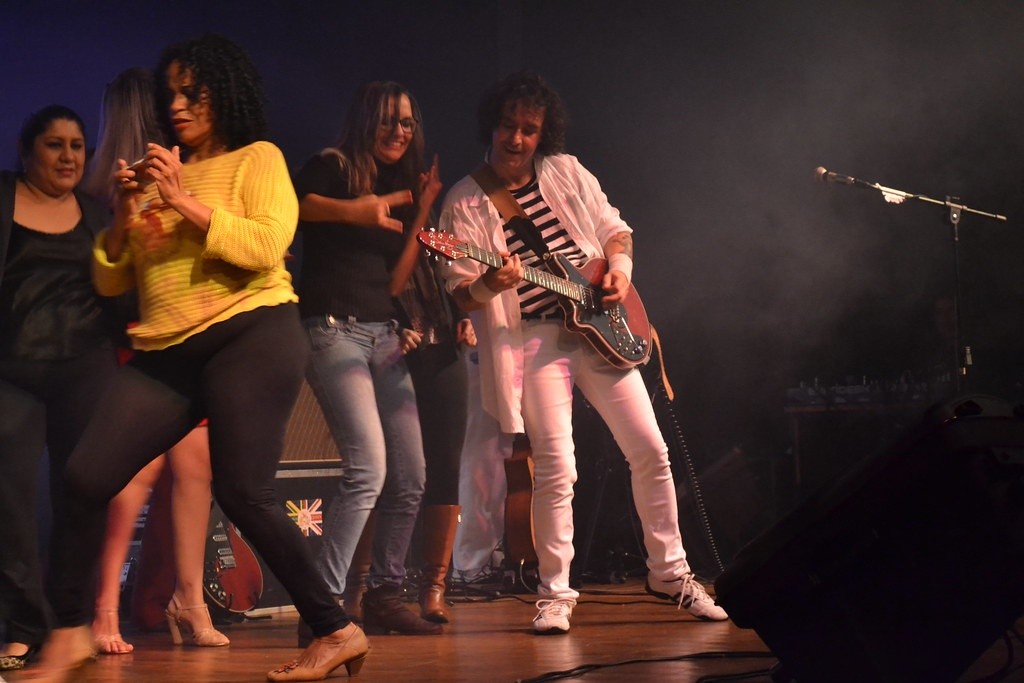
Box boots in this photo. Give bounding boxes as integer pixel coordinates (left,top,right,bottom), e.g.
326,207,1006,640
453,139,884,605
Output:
415,503,464,624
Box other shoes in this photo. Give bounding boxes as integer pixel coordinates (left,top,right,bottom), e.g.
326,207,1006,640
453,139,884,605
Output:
0,643,38,671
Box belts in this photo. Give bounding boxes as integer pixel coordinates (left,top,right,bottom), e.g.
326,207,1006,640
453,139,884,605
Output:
519,313,562,320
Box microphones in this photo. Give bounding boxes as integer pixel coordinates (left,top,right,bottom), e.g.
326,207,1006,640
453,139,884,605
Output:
814,167,855,184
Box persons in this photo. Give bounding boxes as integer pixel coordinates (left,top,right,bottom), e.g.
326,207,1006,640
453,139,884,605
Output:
294,81,448,632
437,70,730,632
1,105,120,668
344,266,479,621
449,346,514,592
0,35,368,682
82,67,228,652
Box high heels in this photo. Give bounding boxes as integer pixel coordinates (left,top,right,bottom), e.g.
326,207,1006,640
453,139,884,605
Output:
164,594,231,647
265,626,371,683
359,583,444,636
90,608,132,654
295,615,313,648
7,652,101,683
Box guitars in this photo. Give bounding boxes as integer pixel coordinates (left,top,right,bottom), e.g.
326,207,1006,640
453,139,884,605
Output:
416,225,651,372
202,517,265,614
504,433,537,565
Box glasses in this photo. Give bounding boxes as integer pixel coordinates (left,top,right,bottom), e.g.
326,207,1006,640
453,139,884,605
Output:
379,115,416,134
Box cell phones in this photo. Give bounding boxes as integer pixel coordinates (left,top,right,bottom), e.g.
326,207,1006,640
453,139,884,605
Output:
124,159,157,187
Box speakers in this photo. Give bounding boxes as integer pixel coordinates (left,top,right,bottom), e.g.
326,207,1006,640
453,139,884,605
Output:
713,392,1024,683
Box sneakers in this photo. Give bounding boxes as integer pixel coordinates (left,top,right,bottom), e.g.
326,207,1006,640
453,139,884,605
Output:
532,596,578,634
644,569,729,621
